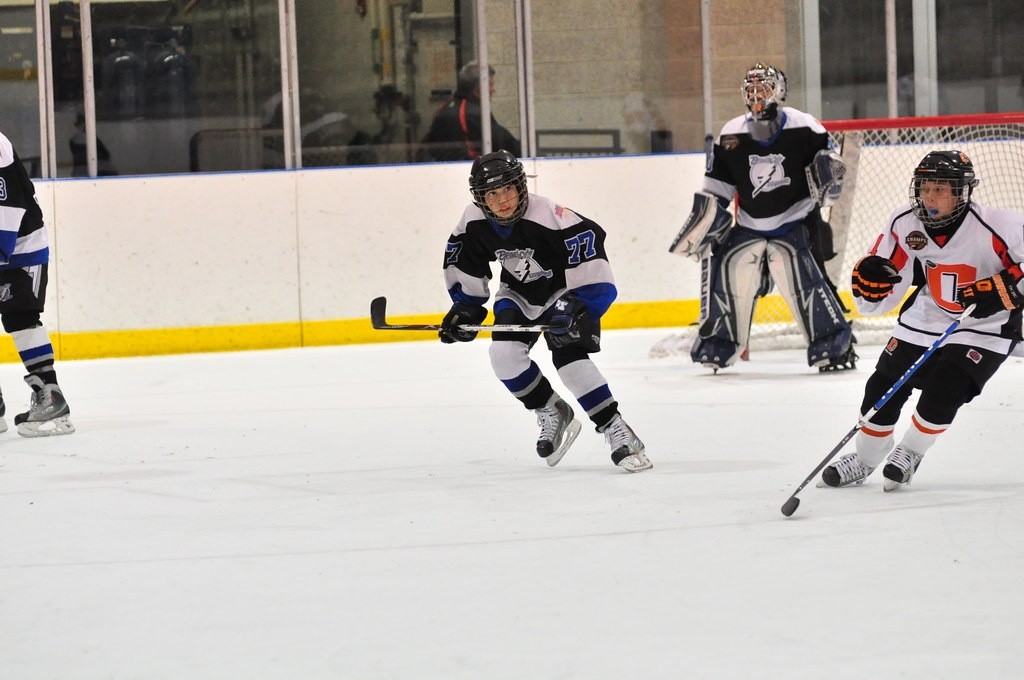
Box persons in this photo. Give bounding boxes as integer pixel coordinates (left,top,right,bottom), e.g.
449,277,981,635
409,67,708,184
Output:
66,112,121,178
349,82,420,165
438,148,652,471
0,129,75,438
815,150,1024,493
415,60,528,161
668,62,859,374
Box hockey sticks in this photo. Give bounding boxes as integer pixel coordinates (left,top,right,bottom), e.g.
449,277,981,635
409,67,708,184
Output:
366,292,553,335
781,304,976,513
686,133,716,328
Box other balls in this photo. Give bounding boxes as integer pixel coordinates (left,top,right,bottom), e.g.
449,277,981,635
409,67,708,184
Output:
783,498,801,517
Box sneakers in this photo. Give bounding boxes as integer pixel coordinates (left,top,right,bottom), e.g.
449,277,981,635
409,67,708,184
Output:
816,453,877,489
14,370,76,437
0,388,8,433
535,391,582,466
595,411,653,472
882,441,924,493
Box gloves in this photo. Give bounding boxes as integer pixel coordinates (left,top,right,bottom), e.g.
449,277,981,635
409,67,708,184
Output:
852,256,902,303
545,295,588,351
438,301,488,343
957,270,1024,319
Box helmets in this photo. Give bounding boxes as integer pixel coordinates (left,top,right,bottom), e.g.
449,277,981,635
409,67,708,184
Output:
914,150,979,228
469,149,529,226
742,61,788,118
